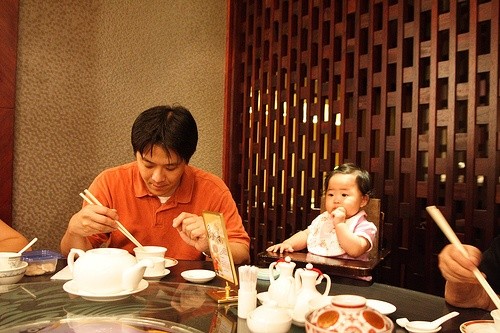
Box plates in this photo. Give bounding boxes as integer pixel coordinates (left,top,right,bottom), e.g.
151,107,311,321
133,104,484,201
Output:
405,321,442,333
256,291,267,305
63,279,149,302
459,320,500,333
142,256,178,280
181,269,216,284
327,296,396,315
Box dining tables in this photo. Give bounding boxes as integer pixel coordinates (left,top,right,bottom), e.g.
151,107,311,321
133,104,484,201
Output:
0,260,494,333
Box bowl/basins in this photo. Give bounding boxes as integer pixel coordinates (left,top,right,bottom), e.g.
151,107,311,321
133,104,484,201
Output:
246,311,293,333
257,268,278,287
0,251,28,285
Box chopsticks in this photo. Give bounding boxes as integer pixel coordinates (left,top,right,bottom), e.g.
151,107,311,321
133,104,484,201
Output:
426,206,500,311
79,189,145,249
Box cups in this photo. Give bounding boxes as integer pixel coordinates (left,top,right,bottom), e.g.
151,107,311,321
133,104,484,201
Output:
237,318,251,333
490,309,500,333
304,294,394,333
132,246,167,275
237,289,257,319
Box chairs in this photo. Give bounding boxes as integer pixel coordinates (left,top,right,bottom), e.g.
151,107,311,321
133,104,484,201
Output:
257,195,390,282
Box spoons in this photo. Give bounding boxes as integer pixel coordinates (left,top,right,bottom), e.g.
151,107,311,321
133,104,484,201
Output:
406,311,459,331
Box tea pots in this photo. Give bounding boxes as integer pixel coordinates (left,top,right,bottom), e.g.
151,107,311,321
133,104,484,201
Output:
266,256,331,321
67,248,154,295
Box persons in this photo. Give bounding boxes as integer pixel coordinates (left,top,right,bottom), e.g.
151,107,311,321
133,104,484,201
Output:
438,243,500,312
266,163,378,281
60,105,250,262
0,218,32,255
